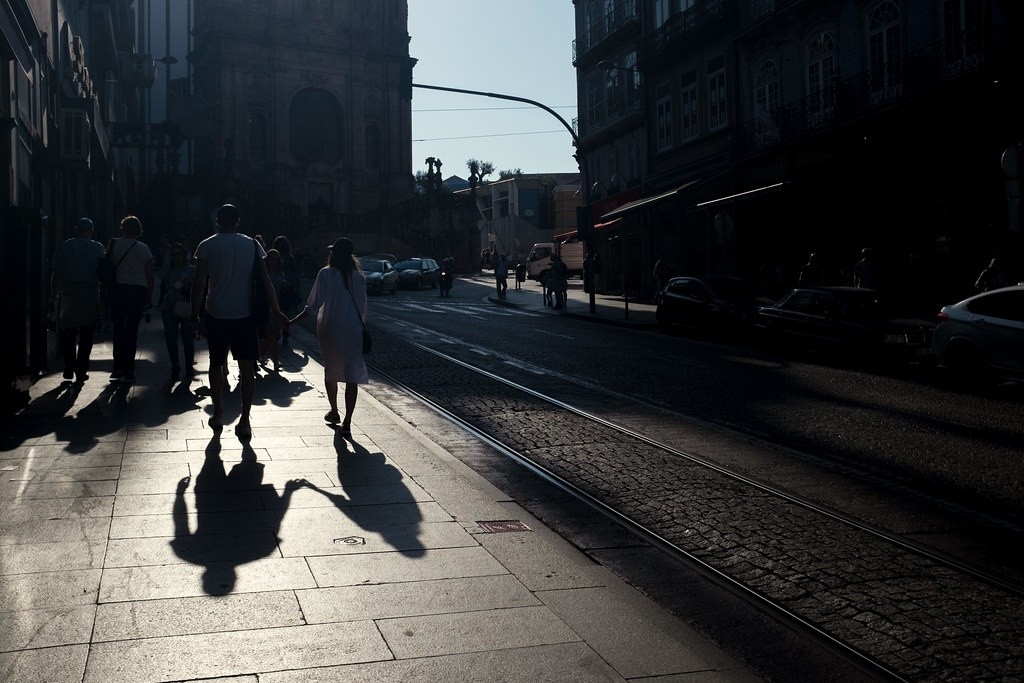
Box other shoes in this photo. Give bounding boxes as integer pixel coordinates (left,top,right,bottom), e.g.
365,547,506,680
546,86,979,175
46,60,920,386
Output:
324,411,341,423
553,304,562,310
185,368,200,377
171,367,181,380
63,370,73,379
208,417,223,431
76,374,89,381
274,364,283,370
235,424,251,444
340,426,351,436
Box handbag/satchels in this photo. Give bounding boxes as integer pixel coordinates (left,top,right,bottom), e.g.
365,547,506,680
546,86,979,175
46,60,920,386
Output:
252,239,270,314
362,328,372,355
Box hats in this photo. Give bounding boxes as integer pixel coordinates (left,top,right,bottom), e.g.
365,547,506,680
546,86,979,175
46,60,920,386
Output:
215,204,240,222
77,217,94,231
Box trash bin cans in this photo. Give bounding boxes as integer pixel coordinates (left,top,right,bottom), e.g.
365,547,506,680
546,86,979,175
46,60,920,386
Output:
516,264,526,282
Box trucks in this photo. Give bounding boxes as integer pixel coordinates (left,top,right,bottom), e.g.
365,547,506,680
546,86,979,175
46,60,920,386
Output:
523,236,584,284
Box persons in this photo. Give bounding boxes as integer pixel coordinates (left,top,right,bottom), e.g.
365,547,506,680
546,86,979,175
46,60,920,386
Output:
436,247,602,310
654,253,675,294
974,256,1004,293
51,203,369,436
799,250,821,292
853,248,876,289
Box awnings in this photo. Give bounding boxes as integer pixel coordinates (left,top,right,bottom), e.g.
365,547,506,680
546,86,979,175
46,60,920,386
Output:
550,171,794,242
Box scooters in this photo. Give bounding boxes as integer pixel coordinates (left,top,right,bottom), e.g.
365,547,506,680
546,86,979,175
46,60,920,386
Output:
434,267,454,297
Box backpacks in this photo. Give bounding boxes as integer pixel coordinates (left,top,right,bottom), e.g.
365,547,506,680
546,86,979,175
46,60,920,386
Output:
96,238,138,293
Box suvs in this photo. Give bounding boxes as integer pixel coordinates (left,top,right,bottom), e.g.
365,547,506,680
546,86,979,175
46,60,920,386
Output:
931,279,1024,392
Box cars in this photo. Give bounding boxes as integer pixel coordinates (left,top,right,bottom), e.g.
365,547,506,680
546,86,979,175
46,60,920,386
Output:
656,273,777,345
368,253,399,266
357,259,401,295
748,286,938,381
394,255,440,291
